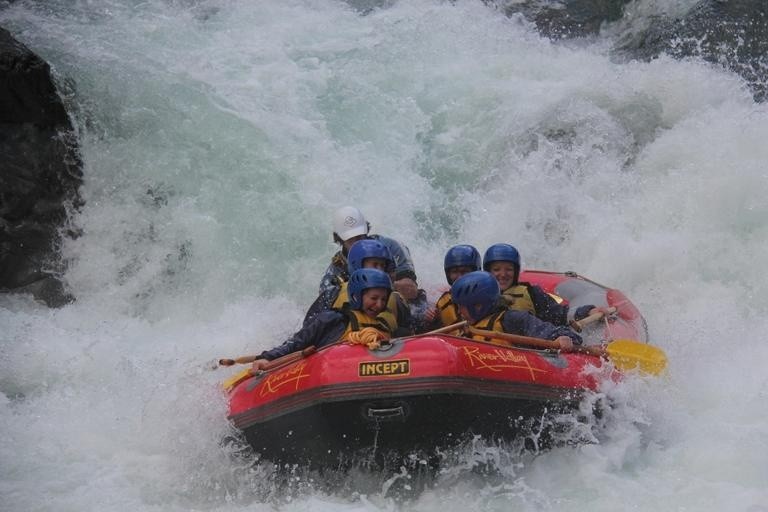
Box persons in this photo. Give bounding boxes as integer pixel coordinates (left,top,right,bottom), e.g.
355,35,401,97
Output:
318,207,418,301
483,243,608,326
414,244,481,338
251,267,417,375
441,271,582,352
302,239,426,328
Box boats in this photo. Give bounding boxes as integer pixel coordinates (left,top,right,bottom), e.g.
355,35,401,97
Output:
223,269,648,498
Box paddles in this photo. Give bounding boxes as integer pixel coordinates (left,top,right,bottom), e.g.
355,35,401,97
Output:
569,289,640,332
222,346,316,390
464,325,666,377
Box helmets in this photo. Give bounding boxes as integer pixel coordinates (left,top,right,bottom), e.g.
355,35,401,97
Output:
445,245,482,286
450,271,500,320
348,268,393,310
483,243,521,284
348,239,395,272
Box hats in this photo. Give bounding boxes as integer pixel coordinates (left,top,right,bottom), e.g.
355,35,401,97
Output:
332,205,368,242
